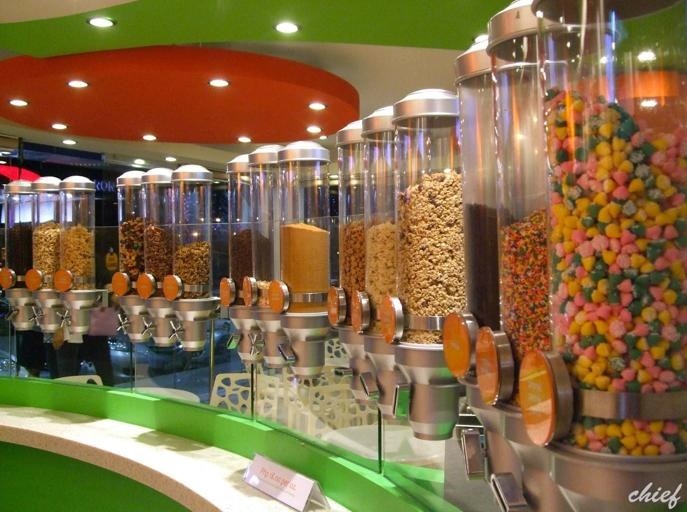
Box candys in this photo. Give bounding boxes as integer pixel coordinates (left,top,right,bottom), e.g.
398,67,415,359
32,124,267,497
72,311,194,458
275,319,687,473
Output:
498,85,687,457
